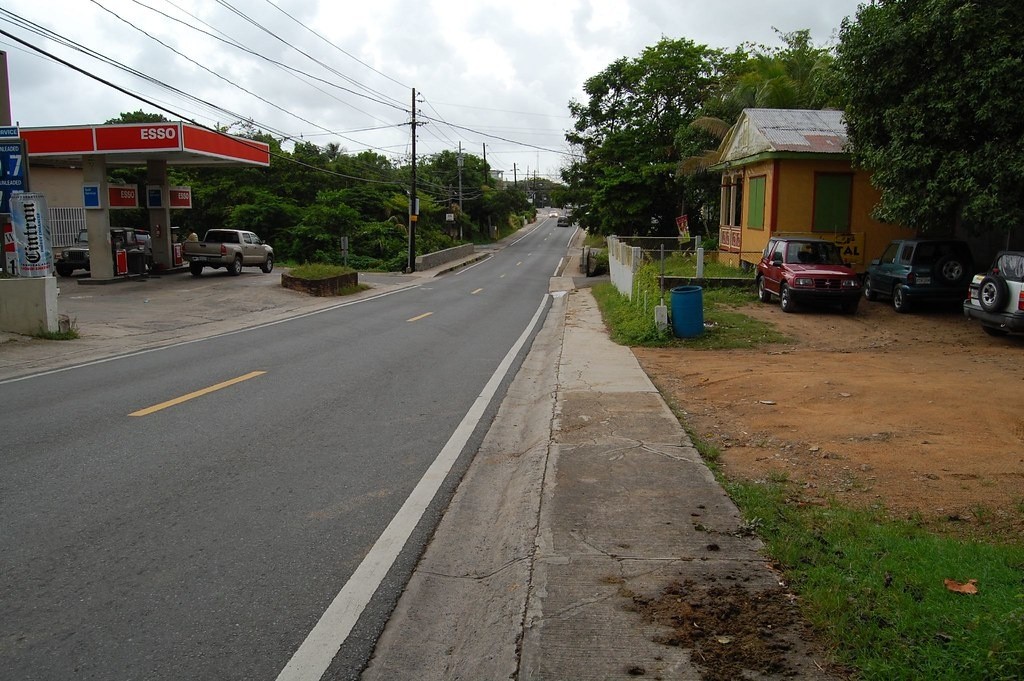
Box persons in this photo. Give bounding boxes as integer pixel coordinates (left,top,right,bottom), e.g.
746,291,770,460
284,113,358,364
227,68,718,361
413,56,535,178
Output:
144,237,153,269
186,228,198,242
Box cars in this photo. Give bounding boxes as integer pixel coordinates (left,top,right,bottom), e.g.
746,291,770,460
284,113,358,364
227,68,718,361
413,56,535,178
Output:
549,211,558,218
566,204,573,208
134,229,151,249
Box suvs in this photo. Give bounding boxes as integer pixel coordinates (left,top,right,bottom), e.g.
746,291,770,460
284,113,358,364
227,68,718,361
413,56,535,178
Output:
864,238,977,314
53,228,139,276
963,249,1024,336
755,237,864,311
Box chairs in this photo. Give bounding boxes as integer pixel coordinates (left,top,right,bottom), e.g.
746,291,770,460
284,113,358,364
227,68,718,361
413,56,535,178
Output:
798,251,814,264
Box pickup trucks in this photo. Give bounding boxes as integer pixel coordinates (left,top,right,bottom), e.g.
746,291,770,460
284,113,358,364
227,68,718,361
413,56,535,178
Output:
183,229,274,276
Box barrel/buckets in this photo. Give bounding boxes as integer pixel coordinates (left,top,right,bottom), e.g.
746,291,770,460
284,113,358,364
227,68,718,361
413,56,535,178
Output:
671,286,704,339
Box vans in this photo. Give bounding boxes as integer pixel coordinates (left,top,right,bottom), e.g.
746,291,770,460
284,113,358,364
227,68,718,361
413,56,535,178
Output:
557,216,570,227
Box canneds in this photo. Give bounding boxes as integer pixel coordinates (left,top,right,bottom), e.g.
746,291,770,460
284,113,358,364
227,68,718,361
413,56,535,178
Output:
8,192,54,278
